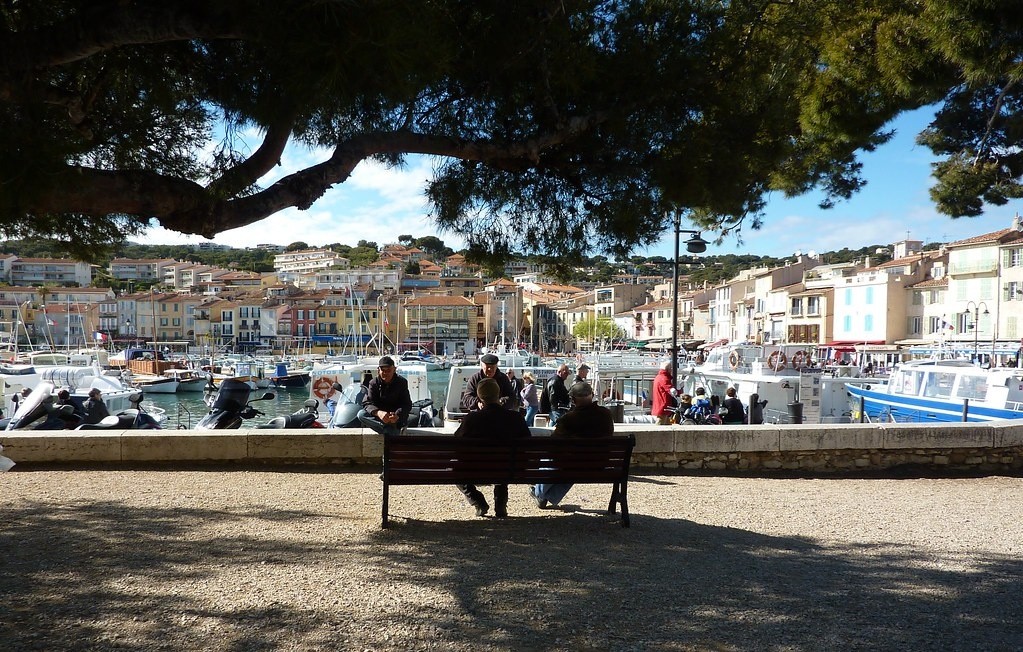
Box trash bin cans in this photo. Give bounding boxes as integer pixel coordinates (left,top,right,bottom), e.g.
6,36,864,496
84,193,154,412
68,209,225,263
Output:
787,402,802,423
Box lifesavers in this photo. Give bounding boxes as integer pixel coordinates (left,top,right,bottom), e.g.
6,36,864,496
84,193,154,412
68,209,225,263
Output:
314,377,336,398
602,389,620,400
728,351,739,370
767,350,787,371
576,354,582,361
792,350,810,371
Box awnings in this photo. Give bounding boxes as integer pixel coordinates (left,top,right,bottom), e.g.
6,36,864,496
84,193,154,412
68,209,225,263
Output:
612,339,728,351
817,341,1021,355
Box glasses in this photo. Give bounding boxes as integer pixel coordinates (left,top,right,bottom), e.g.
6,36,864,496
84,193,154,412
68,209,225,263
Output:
506,372,511,374
523,377,526,379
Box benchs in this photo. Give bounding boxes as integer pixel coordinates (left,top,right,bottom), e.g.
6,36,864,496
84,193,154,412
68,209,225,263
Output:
382,431,634,528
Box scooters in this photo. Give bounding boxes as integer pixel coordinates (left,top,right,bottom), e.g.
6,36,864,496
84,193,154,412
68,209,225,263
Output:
193,373,327,429
327,383,438,427
5,381,169,430
0,382,88,432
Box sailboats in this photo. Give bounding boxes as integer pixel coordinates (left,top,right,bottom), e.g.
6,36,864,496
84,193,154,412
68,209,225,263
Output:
0,271,1023,432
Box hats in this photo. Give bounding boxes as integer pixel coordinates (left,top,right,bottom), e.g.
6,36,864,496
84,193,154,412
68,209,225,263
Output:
727,388,737,397
481,354,499,364
58,390,69,399
557,364,571,373
575,363,591,369
567,381,592,397
379,356,394,366
696,387,706,395
478,378,500,398
88,387,101,397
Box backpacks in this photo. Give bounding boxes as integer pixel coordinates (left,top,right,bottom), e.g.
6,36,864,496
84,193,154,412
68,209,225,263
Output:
539,376,554,413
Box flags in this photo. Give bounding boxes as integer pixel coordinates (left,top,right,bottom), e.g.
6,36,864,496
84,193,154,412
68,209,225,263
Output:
46,315,59,326
942,320,954,330
92,330,108,341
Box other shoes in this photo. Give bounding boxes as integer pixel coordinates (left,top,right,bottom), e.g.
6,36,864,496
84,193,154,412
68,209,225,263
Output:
475,503,489,516
496,509,507,517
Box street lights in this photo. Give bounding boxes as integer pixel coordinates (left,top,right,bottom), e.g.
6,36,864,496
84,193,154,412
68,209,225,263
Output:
752,311,773,344
673,231,712,387
126,319,131,369
251,325,261,356
964,301,990,358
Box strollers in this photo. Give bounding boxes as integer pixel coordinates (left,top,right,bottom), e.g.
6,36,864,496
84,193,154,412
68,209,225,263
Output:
664,395,722,425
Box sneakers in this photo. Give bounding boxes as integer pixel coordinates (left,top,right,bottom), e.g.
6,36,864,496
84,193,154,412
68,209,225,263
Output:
529,486,547,509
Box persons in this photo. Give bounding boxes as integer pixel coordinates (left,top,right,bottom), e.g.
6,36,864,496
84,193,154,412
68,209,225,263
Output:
973,355,1018,368
811,358,871,373
453,349,478,359
539,364,571,428
0,388,110,424
360,370,373,389
463,354,516,412
699,352,704,362
357,356,412,481
520,372,538,428
674,387,768,424
570,363,591,412
447,378,531,518
529,382,614,508
506,368,522,412
651,362,684,425
323,398,338,428
